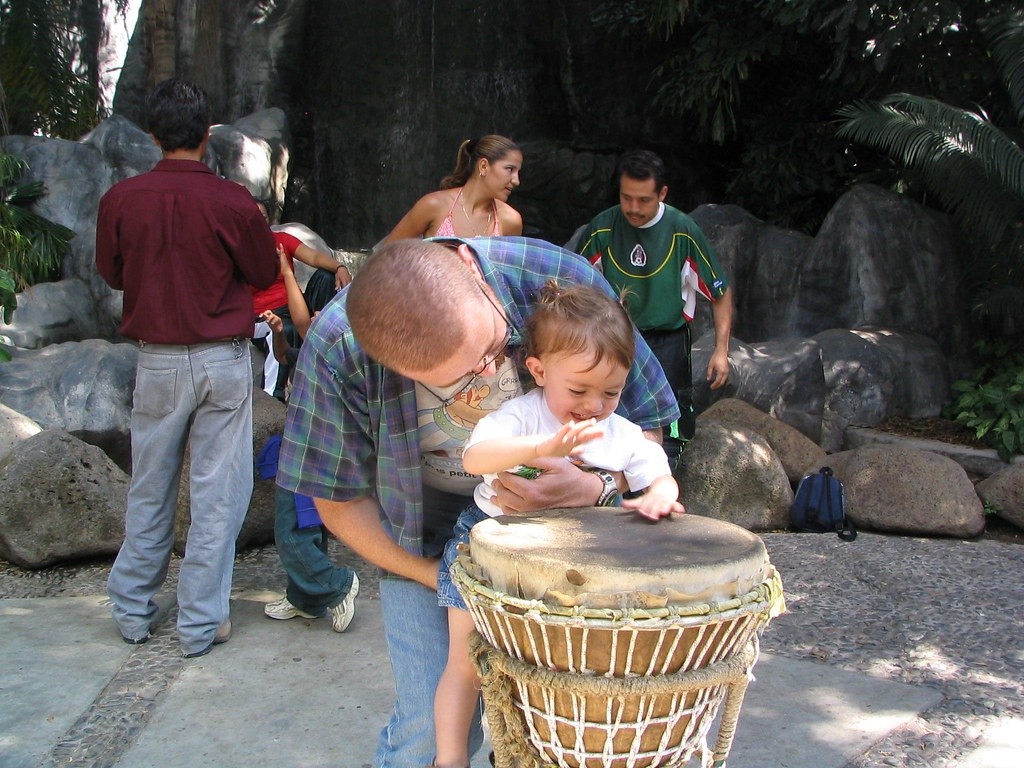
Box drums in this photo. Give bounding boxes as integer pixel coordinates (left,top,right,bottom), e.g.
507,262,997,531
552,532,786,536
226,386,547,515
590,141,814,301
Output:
447,506,781,767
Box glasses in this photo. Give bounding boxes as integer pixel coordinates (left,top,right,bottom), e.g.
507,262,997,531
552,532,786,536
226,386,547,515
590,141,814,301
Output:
416,276,514,406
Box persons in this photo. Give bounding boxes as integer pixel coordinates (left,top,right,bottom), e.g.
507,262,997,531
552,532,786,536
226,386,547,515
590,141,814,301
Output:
381,135,523,248
432,278,684,768
248,200,360,634
97,79,279,657
575,150,733,477
274,235,681,768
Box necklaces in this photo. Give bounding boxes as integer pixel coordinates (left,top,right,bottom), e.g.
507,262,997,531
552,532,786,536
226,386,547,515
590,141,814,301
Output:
461,190,491,238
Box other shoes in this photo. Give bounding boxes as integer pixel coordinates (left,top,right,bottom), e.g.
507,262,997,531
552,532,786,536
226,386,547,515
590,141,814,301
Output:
184,619,232,659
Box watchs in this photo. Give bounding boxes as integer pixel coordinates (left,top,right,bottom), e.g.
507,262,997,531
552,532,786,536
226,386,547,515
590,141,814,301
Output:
588,468,618,507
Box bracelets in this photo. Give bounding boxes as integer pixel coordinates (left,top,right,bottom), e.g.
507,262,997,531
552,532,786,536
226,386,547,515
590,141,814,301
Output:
337,265,346,271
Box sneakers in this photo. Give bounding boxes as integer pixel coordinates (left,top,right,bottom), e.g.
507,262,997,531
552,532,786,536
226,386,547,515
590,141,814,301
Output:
327,571,359,634
264,598,317,619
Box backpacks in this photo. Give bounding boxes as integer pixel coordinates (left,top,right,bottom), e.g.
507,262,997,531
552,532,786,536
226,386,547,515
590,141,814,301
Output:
790,466,857,542
